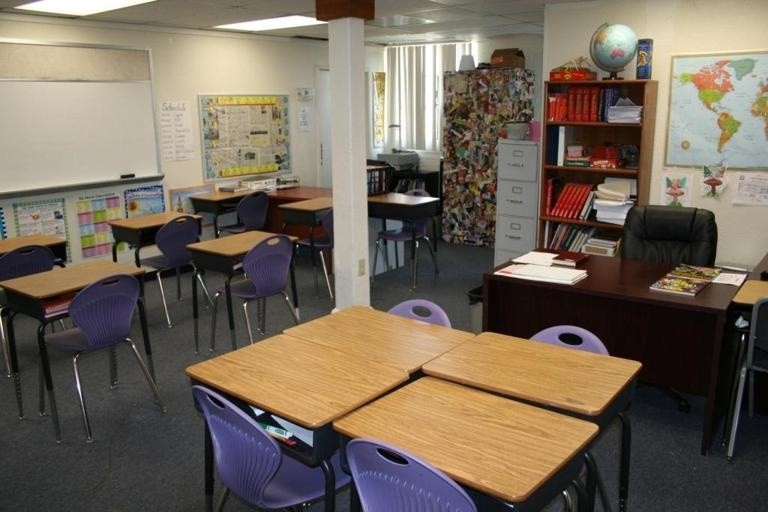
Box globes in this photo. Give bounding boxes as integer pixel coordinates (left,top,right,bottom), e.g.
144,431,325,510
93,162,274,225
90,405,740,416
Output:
589,21,638,80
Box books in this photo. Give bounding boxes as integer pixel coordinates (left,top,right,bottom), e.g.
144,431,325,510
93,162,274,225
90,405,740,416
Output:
552,250,590,267
42,308,69,320
218,174,302,193
648,274,711,296
666,260,723,283
40,291,79,315
367,167,427,197
492,262,588,285
541,83,644,259
513,264,587,282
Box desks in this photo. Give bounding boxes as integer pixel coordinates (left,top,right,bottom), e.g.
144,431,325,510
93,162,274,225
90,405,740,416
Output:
481,250,748,455
732,280,768,308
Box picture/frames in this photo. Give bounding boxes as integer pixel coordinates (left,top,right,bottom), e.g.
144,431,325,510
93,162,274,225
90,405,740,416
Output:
662,50,768,172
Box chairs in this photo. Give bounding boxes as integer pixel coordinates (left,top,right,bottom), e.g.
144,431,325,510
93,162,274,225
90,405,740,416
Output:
724,297,768,460
622,203,720,271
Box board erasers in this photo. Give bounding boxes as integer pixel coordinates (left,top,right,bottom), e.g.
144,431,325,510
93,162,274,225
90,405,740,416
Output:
121,173,134,179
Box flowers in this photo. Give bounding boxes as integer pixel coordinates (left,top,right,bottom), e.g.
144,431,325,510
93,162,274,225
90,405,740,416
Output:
513,90,535,119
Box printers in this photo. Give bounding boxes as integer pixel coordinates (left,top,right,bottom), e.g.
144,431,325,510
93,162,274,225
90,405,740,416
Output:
377,148,419,171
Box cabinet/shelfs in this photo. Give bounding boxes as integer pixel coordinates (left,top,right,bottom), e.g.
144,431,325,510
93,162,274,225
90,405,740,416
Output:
495,139,535,272
538,80,659,253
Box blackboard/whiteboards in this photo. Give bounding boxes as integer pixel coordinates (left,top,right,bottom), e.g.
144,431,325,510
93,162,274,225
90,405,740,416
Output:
0,79,164,199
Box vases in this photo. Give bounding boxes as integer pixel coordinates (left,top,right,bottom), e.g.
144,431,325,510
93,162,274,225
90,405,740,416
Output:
505,120,530,140
